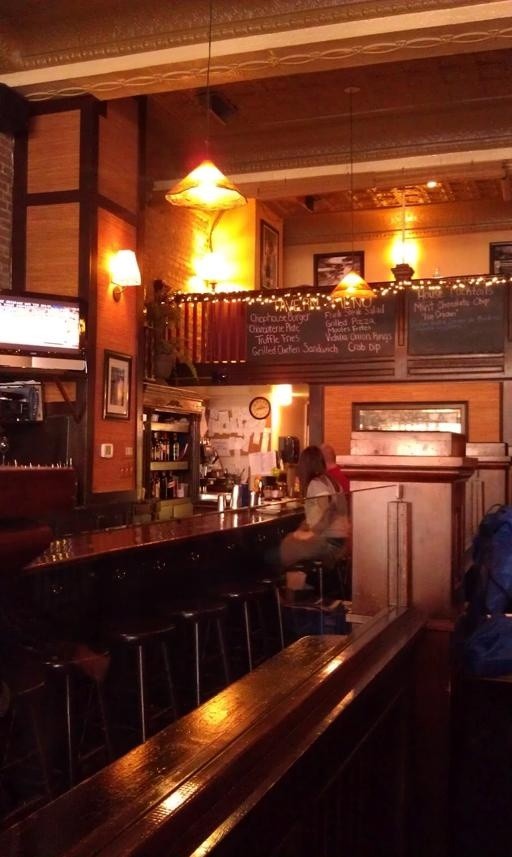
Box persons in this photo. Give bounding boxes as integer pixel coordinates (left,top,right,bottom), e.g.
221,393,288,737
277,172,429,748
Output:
276,445,348,566
319,443,350,493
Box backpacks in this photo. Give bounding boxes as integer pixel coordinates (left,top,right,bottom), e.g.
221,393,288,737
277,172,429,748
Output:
454,502,511,677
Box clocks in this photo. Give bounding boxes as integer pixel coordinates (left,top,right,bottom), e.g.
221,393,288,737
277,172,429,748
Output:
248,396,271,418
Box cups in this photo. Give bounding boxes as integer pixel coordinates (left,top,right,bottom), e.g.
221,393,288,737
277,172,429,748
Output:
215,481,257,513
264,484,281,498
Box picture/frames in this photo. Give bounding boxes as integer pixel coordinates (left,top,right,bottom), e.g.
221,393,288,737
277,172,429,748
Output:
488,240,511,276
312,250,366,286
102,348,133,422
259,219,281,290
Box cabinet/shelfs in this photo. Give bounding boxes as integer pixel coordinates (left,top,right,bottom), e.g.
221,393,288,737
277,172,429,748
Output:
139,381,204,523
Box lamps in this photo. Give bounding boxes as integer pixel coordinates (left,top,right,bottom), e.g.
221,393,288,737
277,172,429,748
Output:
329,86,379,301
163,2,249,214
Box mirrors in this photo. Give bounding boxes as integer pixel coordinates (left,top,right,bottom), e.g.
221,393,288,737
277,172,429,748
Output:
350,401,467,434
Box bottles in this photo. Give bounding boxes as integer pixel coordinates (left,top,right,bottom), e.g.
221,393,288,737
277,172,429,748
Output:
257,480,264,506
151,431,181,498
